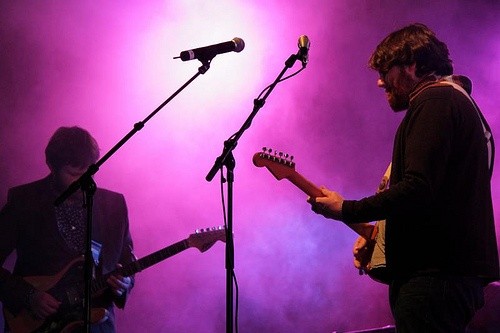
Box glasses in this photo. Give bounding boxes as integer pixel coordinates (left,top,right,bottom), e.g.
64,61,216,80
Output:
379,61,396,81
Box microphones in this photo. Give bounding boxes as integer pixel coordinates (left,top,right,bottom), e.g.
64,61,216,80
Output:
298,35,311,67
172,37,245,63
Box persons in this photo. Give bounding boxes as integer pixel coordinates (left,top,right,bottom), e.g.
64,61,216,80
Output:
1,125,137,333
307,23,500,333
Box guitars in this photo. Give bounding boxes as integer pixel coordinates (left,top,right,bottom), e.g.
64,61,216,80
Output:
253,147,392,285
2,225,233,333
